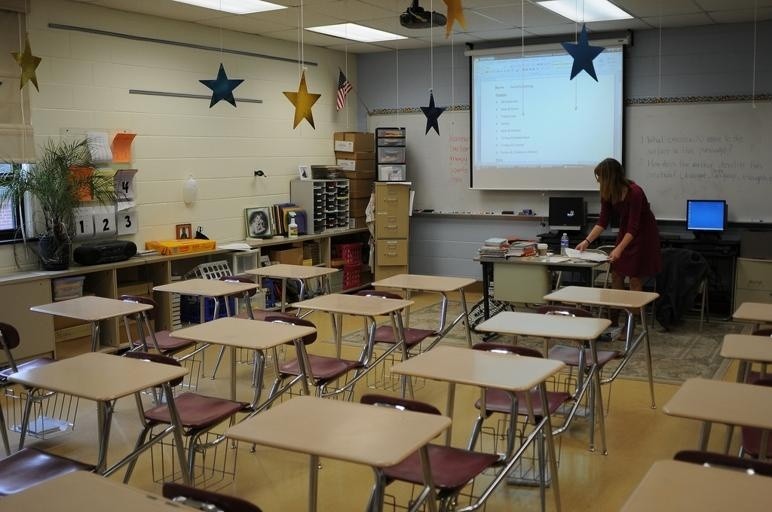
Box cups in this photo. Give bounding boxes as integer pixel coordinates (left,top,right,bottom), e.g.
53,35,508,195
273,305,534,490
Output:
538,244,548,257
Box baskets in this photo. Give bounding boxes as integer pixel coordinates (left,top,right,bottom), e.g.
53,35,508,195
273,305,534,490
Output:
341,265,362,290
183,260,236,306
334,242,363,266
180,297,237,323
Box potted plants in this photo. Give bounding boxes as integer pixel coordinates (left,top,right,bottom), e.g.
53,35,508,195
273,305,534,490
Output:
0,139,119,273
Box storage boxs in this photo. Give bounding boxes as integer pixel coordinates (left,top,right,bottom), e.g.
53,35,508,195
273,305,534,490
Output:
272,246,305,267
334,128,377,226
53,274,159,359
378,124,408,185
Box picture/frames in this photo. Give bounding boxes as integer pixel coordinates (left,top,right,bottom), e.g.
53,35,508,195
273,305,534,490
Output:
176,223,192,239
245,206,275,239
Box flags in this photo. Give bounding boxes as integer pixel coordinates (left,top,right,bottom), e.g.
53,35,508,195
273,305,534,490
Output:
335,71,352,112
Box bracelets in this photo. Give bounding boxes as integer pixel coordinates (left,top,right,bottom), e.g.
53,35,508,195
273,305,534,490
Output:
584,238,590,245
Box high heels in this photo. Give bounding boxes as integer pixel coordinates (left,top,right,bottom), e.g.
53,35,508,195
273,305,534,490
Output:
617,326,634,342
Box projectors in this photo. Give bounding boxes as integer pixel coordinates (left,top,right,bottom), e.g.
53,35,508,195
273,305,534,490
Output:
400,12,447,29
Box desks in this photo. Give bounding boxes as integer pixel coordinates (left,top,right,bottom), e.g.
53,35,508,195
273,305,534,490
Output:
0,469,202,512
225,393,453,509
151,278,259,381
369,273,477,360
475,255,606,342
475,312,615,458
290,292,418,402
662,376,771,467
621,455,770,510
389,345,566,511
241,265,340,343
730,300,772,334
540,230,741,322
719,333,772,384
28,294,155,355
170,316,319,454
7,351,193,492
542,284,661,410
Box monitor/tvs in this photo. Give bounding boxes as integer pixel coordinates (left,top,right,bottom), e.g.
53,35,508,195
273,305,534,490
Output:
686,200,725,235
611,203,650,232
549,197,584,230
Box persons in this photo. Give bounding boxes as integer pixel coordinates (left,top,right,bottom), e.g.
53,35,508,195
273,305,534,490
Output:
249,212,266,236
180,227,188,238
575,158,663,341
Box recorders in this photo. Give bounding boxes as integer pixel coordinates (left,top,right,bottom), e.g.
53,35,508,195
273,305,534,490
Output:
74,241,137,265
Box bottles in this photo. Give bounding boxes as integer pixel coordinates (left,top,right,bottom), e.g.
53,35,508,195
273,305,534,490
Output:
288,218,299,238
560,233,569,257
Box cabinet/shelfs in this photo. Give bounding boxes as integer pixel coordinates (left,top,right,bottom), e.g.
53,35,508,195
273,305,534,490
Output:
331,228,373,290
735,256,772,324
291,179,349,231
115,260,169,344
373,183,414,281
170,245,260,334
260,235,331,296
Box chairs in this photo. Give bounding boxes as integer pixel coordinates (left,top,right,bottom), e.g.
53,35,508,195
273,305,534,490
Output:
118,295,200,386
1,446,100,497
123,351,252,487
353,390,504,512
0,319,62,455
162,480,260,512
537,303,632,416
670,446,770,475
211,276,278,382
249,315,365,453
648,241,715,333
346,290,440,401
466,341,574,488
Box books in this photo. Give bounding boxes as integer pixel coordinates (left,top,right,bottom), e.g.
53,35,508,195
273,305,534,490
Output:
481,238,537,258
